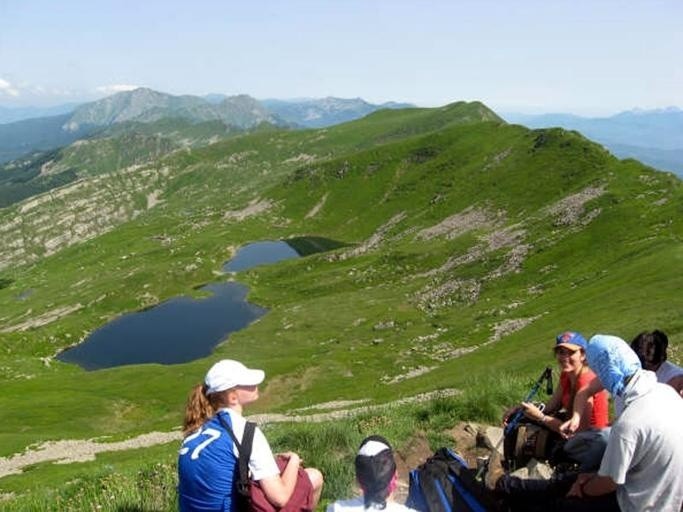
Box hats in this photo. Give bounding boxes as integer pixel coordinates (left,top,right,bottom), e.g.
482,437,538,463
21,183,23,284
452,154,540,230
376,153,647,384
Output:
354,434,397,491
553,331,589,354
204,359,266,396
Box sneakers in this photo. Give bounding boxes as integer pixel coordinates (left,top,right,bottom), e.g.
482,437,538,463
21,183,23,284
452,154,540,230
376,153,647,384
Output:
484,451,503,489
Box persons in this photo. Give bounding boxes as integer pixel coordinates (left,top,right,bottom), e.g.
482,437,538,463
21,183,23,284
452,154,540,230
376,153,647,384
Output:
325,435,417,512
479,334,683,511
557,326,682,444
176,357,324,512
501,330,611,472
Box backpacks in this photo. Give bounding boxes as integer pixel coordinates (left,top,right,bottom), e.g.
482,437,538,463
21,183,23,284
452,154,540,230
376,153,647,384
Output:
405,446,488,511
509,424,545,465
237,422,313,511
